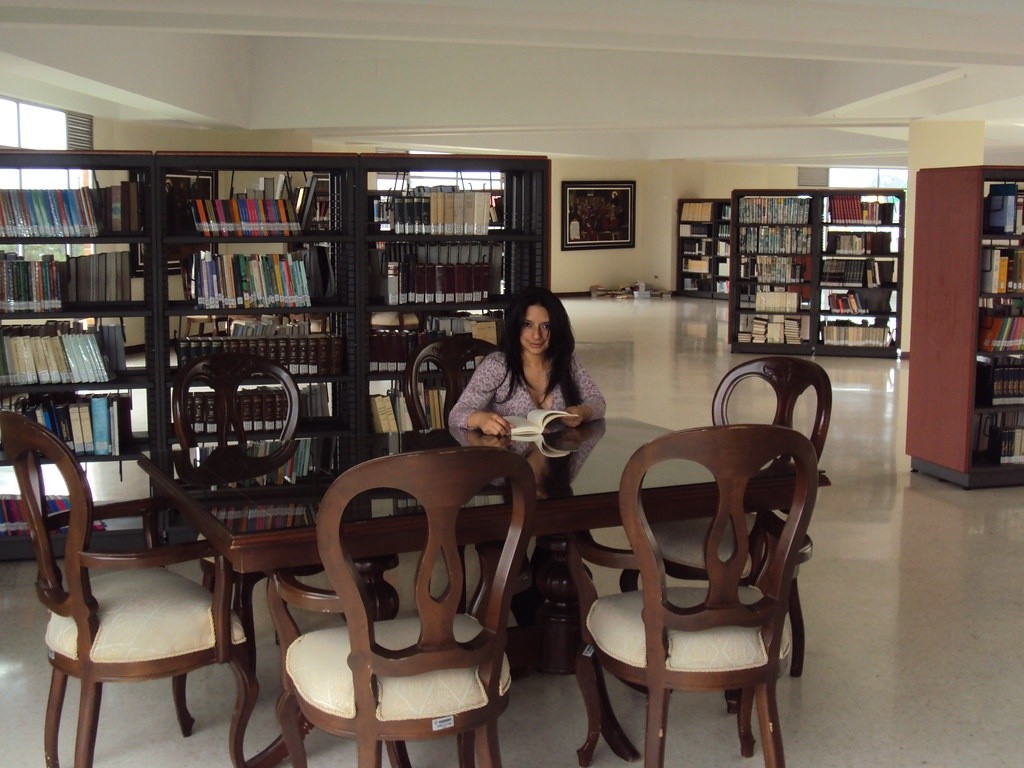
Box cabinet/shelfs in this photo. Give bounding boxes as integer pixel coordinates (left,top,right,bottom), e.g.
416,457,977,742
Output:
815,188,906,362
353,152,553,469
906,164,1024,490
676,197,714,297
0,150,157,561
730,188,817,355
155,149,355,539
714,197,731,300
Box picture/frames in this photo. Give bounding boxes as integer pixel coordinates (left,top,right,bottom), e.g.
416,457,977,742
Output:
560,180,635,251
128,168,219,278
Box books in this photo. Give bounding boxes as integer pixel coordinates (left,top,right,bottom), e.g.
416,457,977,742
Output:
0,321,128,386
737,198,814,344
0,493,106,537
821,193,899,350
0,250,133,314
371,184,506,520
0,180,148,237
972,184,1024,468
510,435,581,457
678,200,732,295
182,171,331,532
499,410,579,435
0,391,132,455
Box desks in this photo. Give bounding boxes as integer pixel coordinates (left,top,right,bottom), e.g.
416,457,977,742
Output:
136,419,833,768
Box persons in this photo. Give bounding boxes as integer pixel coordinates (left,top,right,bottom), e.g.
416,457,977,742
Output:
445,287,607,438
448,419,605,499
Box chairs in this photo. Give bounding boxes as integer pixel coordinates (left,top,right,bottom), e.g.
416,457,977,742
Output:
0,410,258,768
276,446,538,766
173,355,303,609
405,332,497,431
621,358,834,716
575,425,822,766
